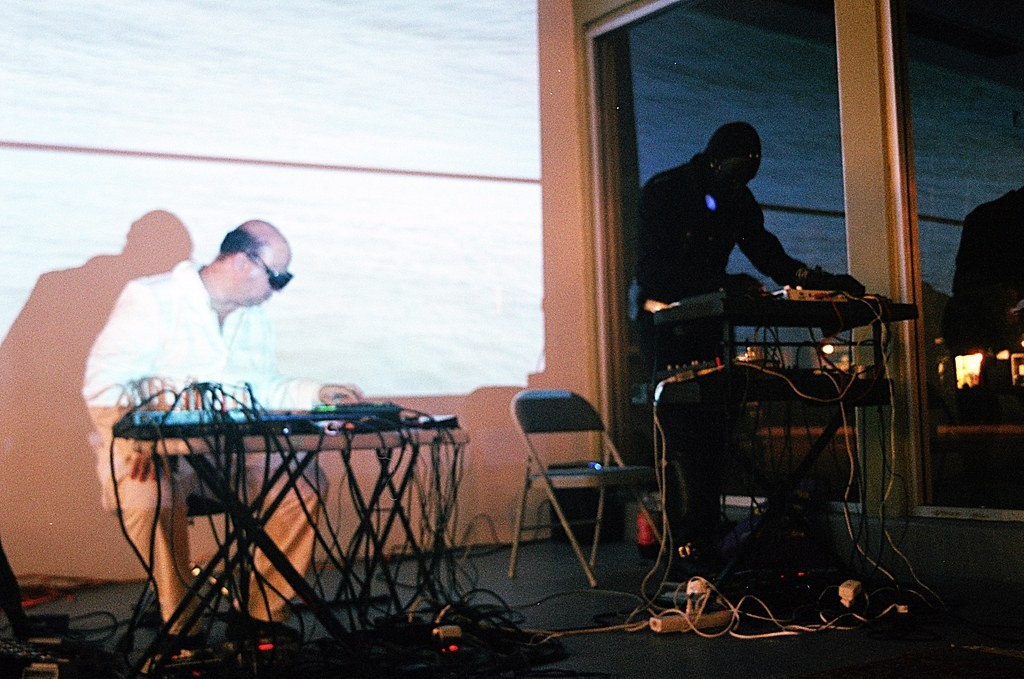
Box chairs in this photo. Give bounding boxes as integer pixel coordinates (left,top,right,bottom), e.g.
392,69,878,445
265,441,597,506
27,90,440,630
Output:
506,388,667,588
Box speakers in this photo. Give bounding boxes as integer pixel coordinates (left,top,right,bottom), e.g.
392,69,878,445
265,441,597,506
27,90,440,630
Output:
549,460,625,544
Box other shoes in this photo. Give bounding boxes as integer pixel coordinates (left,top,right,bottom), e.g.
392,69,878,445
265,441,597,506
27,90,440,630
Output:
226,615,299,646
179,630,214,659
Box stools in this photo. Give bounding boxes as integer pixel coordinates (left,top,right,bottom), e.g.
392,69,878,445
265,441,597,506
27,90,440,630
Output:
114,493,248,659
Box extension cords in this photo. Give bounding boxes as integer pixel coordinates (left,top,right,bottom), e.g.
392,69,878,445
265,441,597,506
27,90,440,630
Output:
649,610,731,633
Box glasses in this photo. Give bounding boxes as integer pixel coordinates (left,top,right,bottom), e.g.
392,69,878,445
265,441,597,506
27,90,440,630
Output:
247,252,293,291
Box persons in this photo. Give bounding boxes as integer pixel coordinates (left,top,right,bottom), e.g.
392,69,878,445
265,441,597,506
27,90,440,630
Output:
80,218,366,659
630,110,870,590
936,179,1024,507
